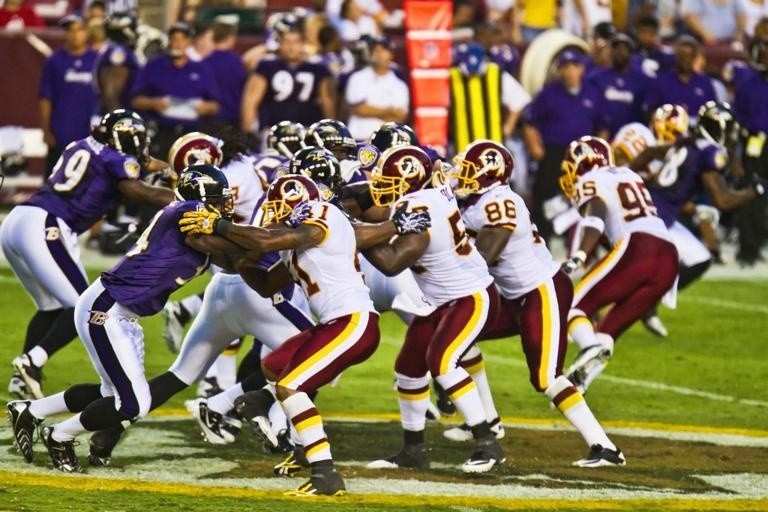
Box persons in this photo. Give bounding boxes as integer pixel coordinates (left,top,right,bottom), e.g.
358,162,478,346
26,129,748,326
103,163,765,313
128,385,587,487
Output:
1,1,768,494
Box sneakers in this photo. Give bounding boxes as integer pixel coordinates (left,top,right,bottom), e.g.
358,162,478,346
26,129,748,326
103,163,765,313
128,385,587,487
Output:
87,430,114,468
232,390,280,450
200,416,244,444
39,423,87,474
363,446,434,473
6,349,50,401
273,446,312,477
422,395,459,420
197,378,225,398
442,415,508,443
564,343,614,399
459,440,508,474
6,397,44,464
640,309,671,339
281,471,350,498
182,396,227,446
571,442,629,470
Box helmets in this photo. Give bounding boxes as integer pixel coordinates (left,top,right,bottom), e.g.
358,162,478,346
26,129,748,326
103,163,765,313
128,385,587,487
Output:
54,6,396,54
302,118,359,153
290,144,345,188
697,99,737,145
168,130,226,177
174,164,233,207
375,143,436,203
94,105,151,156
369,119,422,153
454,137,516,194
266,119,306,151
652,103,691,144
564,134,617,178
266,173,323,219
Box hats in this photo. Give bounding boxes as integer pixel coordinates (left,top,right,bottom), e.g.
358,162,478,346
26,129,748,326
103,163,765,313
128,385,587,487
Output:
556,23,698,67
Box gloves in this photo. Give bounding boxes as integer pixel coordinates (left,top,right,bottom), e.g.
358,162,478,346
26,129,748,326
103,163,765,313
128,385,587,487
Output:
390,201,433,236
177,204,222,238
560,255,584,276
